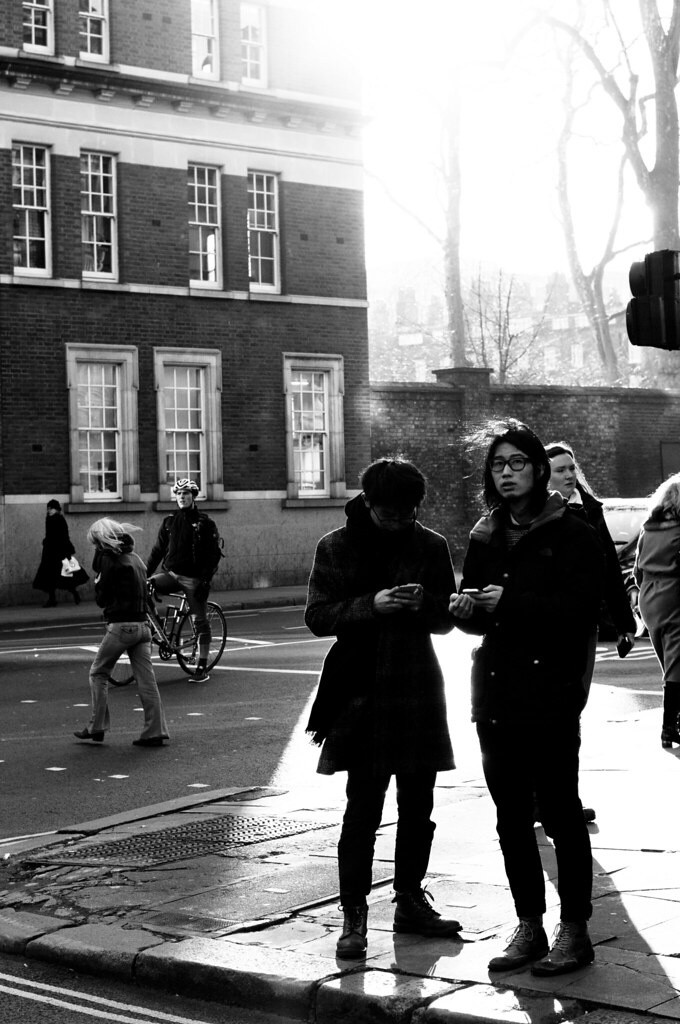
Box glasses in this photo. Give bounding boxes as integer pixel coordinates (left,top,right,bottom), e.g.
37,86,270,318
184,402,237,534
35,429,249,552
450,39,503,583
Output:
487,457,530,472
370,506,418,526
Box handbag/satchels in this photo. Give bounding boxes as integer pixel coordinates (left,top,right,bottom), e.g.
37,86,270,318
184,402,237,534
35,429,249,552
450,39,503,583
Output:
60,556,81,577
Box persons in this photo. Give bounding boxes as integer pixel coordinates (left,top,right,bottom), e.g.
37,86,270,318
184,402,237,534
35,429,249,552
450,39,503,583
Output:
634,473,680,748
147,478,221,683
73,518,170,747
33,500,90,608
303,459,463,958
448,419,602,977
538,441,636,825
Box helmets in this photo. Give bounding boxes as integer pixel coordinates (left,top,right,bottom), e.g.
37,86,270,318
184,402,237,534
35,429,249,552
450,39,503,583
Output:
173,479,199,496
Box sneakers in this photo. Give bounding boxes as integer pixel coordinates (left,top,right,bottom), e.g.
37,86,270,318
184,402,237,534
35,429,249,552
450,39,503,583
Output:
488,917,549,972
532,922,595,975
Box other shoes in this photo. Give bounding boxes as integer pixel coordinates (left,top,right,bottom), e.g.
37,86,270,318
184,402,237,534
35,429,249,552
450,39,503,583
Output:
584,808,596,821
188,671,210,682
42,601,57,608
73,728,104,744
73,591,80,605
133,738,161,747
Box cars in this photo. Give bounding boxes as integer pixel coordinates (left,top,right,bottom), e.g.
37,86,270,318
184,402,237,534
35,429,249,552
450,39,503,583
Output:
597,495,654,640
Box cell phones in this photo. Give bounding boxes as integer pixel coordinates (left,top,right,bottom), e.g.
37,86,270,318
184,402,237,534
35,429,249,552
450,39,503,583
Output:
463,589,486,595
617,635,633,658
388,584,417,597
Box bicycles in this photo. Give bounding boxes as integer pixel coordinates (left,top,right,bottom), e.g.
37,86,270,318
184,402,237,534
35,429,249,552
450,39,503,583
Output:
106,578,228,688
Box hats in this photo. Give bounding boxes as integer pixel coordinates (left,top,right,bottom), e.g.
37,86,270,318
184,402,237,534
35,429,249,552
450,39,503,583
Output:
47,500,61,511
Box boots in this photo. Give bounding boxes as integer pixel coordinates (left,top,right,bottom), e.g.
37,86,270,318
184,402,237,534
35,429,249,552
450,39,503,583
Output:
337,904,369,955
661,681,680,748
391,885,463,936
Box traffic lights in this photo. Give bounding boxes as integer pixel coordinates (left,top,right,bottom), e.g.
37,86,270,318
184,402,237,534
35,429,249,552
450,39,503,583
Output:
625,249,679,352
